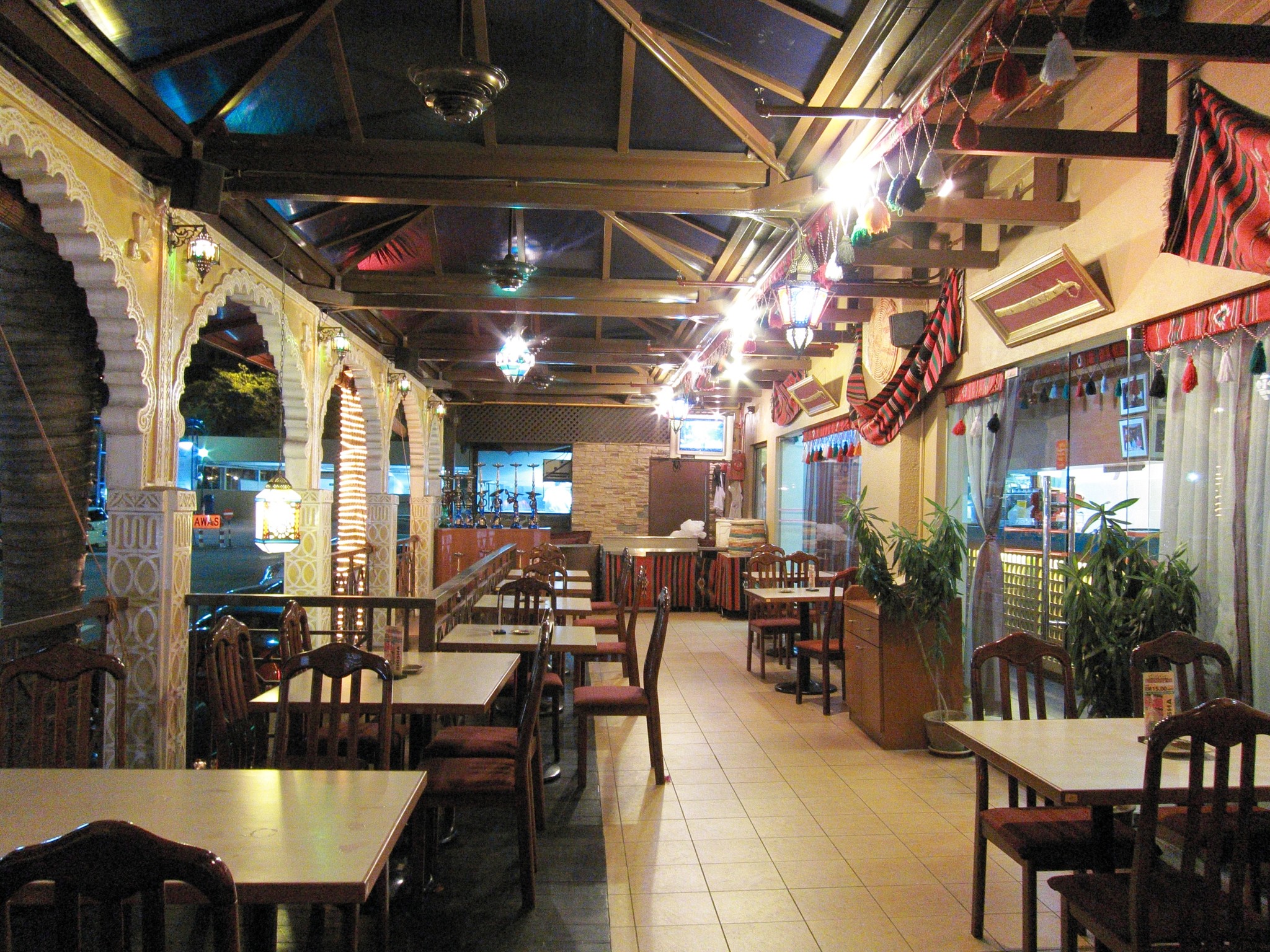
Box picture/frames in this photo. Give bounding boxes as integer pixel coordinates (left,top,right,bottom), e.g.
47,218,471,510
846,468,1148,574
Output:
966,242,1114,349
786,374,839,417
1118,371,1153,459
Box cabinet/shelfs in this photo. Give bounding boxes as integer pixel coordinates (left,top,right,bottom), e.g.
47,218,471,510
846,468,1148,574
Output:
841,585,962,752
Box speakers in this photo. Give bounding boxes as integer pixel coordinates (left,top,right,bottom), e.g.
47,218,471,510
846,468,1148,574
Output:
888,310,926,345
171,155,221,214
394,347,420,371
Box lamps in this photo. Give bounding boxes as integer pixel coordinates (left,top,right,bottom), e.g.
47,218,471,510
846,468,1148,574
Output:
253,236,302,555
166,212,447,424
771,222,830,363
406,0,554,390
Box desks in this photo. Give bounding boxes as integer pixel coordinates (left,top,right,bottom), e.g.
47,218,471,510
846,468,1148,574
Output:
507,569,590,582
742,571,834,656
241,648,520,906
475,592,591,719
2,768,428,950
745,586,849,694
494,579,592,599
443,624,597,788
940,718,1270,952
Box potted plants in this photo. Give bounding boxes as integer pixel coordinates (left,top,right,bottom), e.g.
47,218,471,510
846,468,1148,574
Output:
837,486,974,756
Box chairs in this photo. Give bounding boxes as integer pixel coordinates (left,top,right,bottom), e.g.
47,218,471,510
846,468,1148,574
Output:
970,633,1266,952
745,544,861,716
0,548,669,950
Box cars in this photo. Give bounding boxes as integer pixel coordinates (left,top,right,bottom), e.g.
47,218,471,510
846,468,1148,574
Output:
84,506,109,549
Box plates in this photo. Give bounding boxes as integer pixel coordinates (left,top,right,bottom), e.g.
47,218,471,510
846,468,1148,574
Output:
532,599,546,602
1163,740,1213,756
511,629,531,634
780,589,794,592
402,665,424,675
